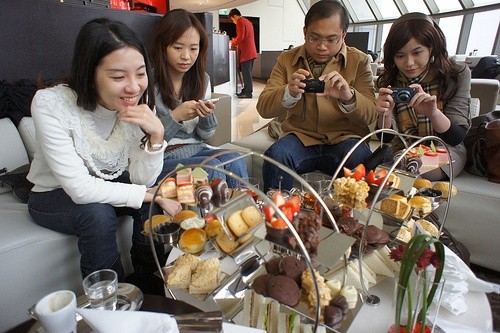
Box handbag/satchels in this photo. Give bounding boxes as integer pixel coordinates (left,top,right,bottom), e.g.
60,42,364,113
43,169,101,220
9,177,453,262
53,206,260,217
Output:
464,110,500,181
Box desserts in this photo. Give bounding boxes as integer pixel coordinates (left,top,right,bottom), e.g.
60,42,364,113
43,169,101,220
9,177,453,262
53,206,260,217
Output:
253,256,358,330
330,141,458,245
144,163,320,296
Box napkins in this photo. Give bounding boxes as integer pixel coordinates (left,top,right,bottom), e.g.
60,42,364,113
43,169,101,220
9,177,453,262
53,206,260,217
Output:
73,307,180,333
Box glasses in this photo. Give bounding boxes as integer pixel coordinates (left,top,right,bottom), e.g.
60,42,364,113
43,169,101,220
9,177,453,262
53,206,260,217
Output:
305,27,344,46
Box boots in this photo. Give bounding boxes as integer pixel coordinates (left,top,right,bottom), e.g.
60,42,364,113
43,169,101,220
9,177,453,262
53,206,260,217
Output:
412,211,470,266
81,255,124,283
130,236,166,296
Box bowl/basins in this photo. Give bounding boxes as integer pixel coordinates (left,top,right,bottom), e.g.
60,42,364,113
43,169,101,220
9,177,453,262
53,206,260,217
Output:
153,220,181,244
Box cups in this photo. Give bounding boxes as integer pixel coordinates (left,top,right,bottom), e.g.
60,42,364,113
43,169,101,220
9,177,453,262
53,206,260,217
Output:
235,176,260,204
300,172,331,210
35,290,77,333
83,269,118,311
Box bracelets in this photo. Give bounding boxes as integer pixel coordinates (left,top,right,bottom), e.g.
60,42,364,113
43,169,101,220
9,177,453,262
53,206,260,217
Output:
149,143,162,150
341,88,356,105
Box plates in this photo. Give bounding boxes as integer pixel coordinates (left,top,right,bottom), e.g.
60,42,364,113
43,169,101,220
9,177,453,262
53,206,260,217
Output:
30,282,143,333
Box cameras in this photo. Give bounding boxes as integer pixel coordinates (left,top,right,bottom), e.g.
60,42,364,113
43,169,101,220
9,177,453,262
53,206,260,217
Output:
388,87,415,105
299,80,325,93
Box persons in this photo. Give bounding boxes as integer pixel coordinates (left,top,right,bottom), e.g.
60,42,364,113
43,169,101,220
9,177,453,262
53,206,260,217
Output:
26,19,168,283
148,8,250,189
229,8,258,98
257,0,380,196
374,11,471,182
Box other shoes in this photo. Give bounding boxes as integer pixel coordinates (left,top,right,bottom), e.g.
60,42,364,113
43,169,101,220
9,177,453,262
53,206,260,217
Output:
237,92,252,98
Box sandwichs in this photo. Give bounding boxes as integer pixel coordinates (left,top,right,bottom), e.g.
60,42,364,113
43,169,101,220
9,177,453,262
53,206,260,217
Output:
242,288,327,333
323,243,402,290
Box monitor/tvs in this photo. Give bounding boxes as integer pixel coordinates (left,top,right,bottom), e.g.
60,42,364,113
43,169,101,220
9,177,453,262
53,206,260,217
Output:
0,0,165,126
345,32,369,53
220,14,260,52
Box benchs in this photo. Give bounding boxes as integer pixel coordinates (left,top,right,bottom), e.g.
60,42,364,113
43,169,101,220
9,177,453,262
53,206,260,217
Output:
232,127,500,272
0,184,133,333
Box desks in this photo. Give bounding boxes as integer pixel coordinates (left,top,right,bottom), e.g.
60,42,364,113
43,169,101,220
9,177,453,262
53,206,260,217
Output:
163,202,493,333
75,294,221,333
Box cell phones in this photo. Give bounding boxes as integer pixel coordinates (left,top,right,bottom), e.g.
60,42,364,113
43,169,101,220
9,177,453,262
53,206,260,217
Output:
204,98,219,108
